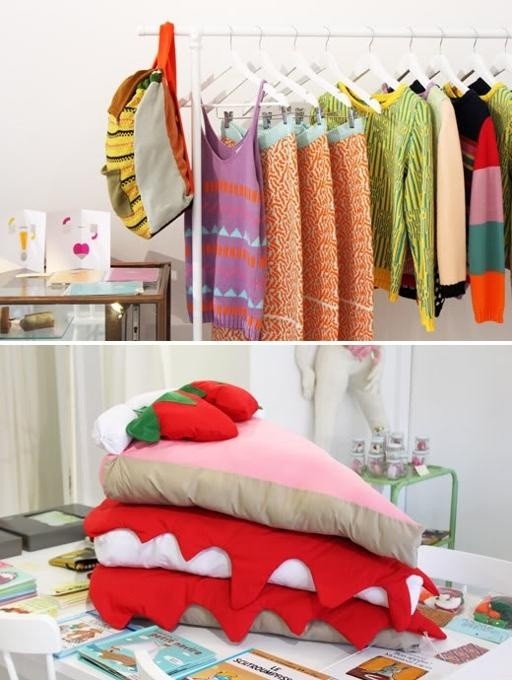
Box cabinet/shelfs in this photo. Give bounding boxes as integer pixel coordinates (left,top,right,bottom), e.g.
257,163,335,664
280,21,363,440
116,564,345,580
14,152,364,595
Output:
357,462,456,552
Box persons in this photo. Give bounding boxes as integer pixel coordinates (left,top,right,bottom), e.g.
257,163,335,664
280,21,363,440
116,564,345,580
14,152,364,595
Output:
295,344,390,454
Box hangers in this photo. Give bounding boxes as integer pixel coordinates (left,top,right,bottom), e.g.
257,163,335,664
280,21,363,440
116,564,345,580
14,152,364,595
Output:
175,24,510,125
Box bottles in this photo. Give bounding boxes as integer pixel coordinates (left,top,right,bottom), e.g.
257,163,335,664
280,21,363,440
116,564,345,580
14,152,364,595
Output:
349,432,430,481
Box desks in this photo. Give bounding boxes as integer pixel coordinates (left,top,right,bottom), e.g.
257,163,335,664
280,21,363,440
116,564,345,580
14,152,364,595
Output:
1,259,174,343
0,539,512,680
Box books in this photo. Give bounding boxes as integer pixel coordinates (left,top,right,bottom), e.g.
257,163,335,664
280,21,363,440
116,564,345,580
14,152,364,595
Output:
63,281,146,296
48,269,104,285
107,267,160,285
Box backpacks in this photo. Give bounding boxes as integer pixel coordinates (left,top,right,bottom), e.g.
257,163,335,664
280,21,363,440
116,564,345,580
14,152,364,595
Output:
101,21,194,244
